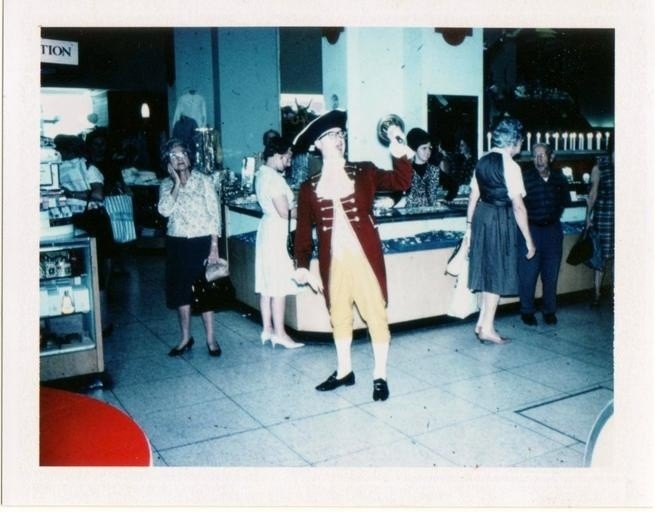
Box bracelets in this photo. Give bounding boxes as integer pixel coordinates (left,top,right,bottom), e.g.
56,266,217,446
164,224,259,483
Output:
466,220,472,224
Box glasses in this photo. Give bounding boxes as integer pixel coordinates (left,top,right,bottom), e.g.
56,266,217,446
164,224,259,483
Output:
169,151,187,158
318,131,346,140
533,151,546,158
419,145,433,151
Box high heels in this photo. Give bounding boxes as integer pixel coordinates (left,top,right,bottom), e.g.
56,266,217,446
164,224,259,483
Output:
168,335,194,357
261,331,272,345
270,334,305,349
207,338,222,356
475,324,512,344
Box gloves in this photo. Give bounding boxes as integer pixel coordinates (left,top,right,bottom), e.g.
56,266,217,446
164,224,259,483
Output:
387,125,406,158
292,268,310,285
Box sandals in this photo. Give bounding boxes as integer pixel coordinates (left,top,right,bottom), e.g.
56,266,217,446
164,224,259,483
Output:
591,299,600,310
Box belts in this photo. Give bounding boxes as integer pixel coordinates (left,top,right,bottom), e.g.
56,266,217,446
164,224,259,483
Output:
482,197,512,207
529,218,558,226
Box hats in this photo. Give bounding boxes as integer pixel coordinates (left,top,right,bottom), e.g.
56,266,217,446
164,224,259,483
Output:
292,109,346,152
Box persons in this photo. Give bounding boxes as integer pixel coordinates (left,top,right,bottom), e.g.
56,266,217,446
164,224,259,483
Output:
55,127,128,335
391,131,460,210
436,137,461,200
293,124,414,402
459,120,536,345
518,143,572,326
460,133,477,184
587,134,614,308
261,130,291,180
157,138,223,358
254,137,306,350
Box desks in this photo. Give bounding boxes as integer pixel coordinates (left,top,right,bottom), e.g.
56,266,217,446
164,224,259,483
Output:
40,388,152,466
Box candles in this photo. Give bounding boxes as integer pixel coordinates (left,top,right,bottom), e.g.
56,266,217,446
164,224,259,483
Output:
588,133,593,150
605,131,610,149
570,133,573,151
597,132,600,150
527,132,531,152
555,132,559,152
487,133,491,151
574,133,576,151
546,133,550,144
564,132,567,150
579,133,584,150
537,132,541,143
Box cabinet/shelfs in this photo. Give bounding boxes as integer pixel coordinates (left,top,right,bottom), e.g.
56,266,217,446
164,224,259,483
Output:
219,192,613,341
39,230,104,391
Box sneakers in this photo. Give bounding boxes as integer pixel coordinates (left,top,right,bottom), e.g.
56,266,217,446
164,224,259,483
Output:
543,313,557,324
521,313,538,325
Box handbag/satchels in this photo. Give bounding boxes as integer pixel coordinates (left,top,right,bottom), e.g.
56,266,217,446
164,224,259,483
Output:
287,230,314,259
567,238,593,265
445,238,477,319
205,257,229,282
583,230,602,272
104,194,137,244
192,275,240,314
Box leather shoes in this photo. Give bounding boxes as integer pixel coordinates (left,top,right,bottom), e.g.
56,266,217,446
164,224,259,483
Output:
316,371,355,391
373,378,388,401
